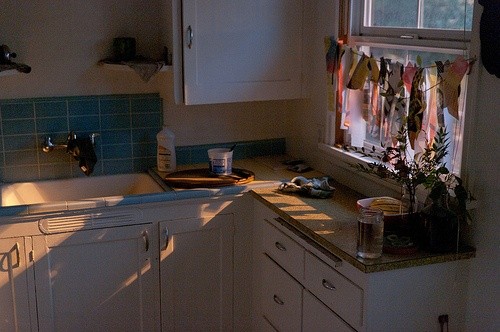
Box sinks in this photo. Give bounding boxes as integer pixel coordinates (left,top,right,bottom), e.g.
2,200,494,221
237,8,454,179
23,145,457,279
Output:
0,172,168,214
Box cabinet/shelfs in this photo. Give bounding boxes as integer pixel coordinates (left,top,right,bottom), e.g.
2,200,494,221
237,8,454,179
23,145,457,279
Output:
250,218,367,332
158,1,312,106
31,213,235,332
0,236,38,332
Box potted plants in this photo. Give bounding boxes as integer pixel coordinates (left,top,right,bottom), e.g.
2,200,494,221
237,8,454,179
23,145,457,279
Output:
352,126,461,257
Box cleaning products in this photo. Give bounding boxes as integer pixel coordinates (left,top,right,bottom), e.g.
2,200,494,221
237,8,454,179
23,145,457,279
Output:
156,124,177,172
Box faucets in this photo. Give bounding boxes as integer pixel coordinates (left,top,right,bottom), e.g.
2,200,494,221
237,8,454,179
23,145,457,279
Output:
66,130,77,150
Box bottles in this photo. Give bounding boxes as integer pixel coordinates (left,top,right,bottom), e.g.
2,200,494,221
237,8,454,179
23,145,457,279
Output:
156,124,176,173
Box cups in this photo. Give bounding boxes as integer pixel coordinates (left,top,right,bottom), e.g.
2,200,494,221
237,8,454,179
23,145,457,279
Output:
207,147,234,176
358,206,384,260
111,36,136,64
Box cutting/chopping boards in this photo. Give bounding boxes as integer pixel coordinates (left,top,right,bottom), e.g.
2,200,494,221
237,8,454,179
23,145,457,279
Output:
165,167,255,188
